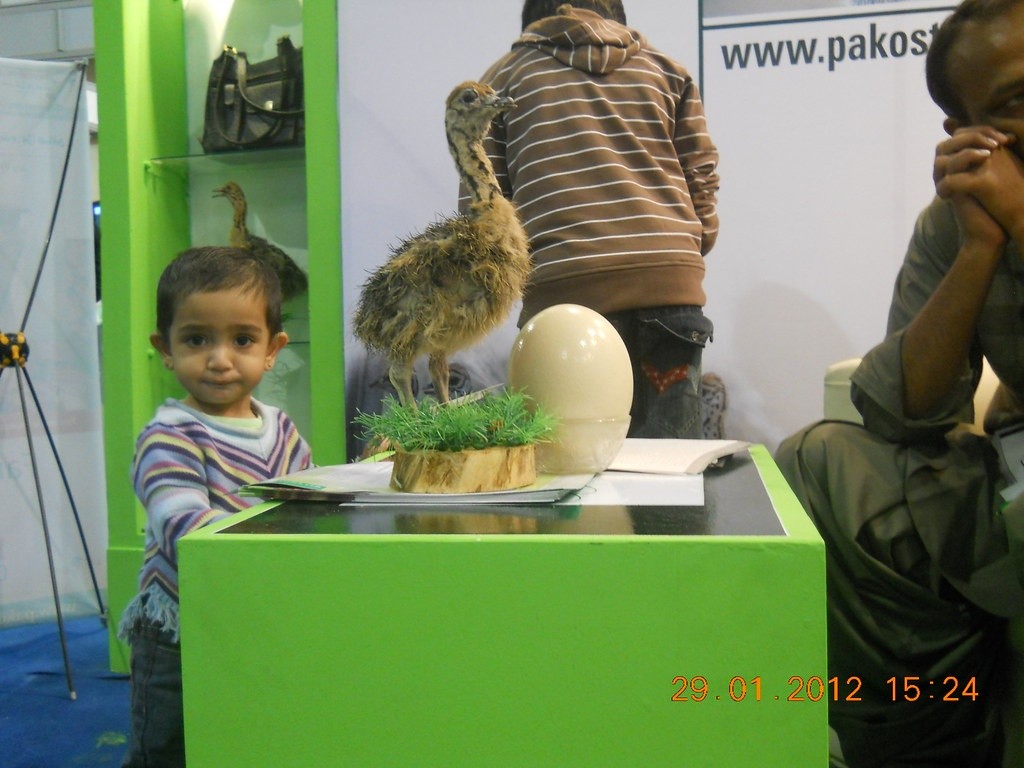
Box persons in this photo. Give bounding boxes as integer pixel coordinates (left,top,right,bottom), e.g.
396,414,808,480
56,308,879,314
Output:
772,0,1024,768
458,0,721,441
119,242,318,768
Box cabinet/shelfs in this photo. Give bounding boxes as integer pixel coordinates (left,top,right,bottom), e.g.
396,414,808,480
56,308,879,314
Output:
91,1,348,674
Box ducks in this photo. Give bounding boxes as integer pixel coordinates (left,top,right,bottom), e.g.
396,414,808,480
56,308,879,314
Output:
422,363,473,399
211,182,307,299
367,365,419,407
351,81,530,412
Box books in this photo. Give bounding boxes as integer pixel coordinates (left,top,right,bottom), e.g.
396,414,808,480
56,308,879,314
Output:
224,468,706,508
505,437,755,475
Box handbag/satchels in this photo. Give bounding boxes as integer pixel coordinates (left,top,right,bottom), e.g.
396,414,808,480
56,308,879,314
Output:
200,34,305,147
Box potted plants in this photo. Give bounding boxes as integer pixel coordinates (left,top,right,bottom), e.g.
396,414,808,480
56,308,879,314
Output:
350,391,563,491
